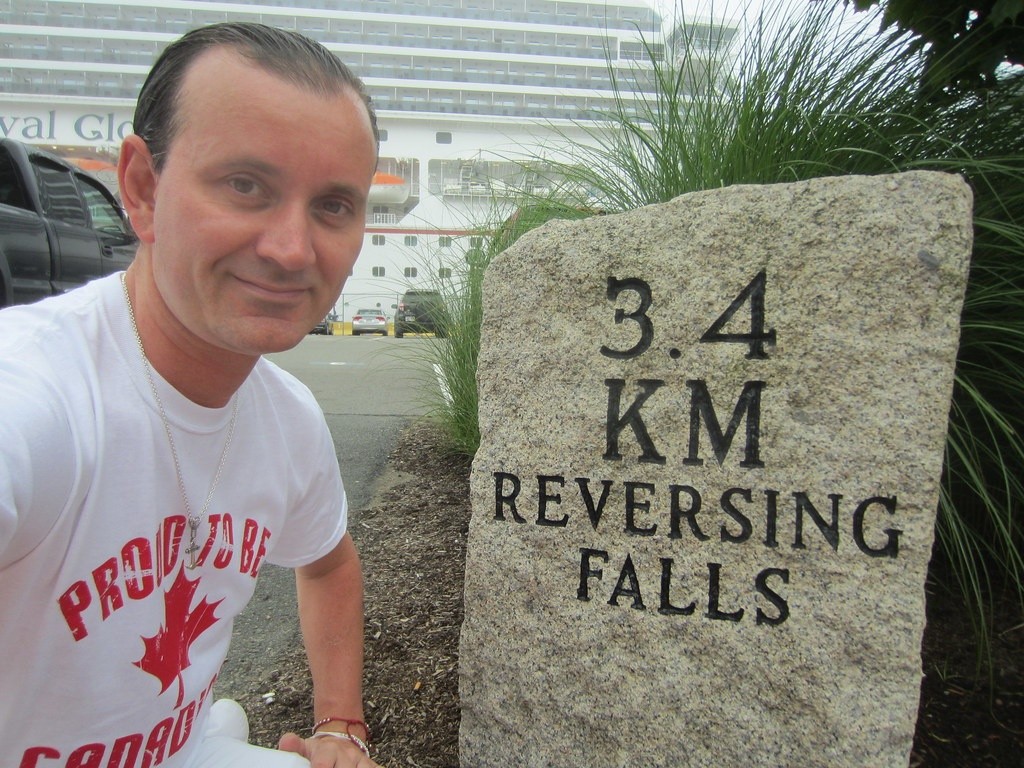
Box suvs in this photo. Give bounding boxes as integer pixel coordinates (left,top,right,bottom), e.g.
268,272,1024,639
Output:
392,289,449,338
0,138,141,310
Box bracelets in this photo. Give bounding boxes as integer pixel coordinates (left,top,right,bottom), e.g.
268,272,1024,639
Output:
313,718,371,758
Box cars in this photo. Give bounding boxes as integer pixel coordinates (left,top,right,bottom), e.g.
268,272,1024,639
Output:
309,313,334,335
351,309,391,336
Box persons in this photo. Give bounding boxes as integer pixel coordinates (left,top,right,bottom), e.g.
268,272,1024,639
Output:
1,20,384,768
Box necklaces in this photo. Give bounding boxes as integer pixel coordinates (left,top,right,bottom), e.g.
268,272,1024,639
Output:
121,271,238,570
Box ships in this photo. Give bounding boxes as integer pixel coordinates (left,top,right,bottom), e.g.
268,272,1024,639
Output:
0,0,742,320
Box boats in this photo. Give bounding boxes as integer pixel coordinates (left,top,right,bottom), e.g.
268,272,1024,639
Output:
60,155,121,195
367,169,409,205
